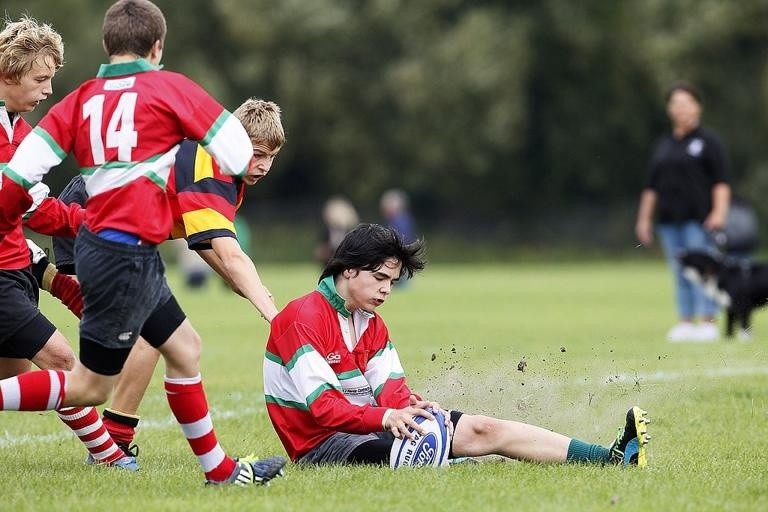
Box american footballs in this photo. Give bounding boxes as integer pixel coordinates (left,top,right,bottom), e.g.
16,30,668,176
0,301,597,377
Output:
389,409,451,466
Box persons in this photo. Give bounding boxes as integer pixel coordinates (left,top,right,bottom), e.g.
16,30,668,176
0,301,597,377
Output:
371,193,419,285
314,199,361,265
0,1,286,488
0,16,137,473
19,101,284,456
261,225,652,474
637,81,731,343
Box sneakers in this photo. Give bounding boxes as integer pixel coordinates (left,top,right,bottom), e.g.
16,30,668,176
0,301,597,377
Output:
609,406,652,467
204,455,288,489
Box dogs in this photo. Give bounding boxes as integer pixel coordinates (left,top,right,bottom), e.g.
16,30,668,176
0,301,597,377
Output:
677,246,768,339
